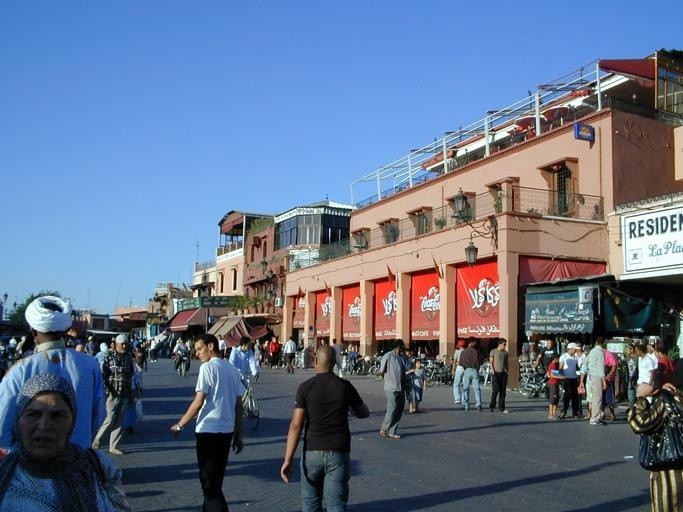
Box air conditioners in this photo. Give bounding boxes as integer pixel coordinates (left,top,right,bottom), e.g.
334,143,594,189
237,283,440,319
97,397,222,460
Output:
275,297,282,307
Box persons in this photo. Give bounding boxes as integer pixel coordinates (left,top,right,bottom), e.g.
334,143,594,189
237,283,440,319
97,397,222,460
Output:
0,295,107,448
280,344,368,512
0,324,157,440
624,357,682,511
379,339,425,440
91,334,134,454
169,333,246,512
0,374,129,512
452,336,512,413
626,341,672,406
532,338,619,426
173,335,301,420
318,338,359,379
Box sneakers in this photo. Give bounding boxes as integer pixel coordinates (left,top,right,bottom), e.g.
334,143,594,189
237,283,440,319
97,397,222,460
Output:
107,447,124,455
91,436,101,449
379,429,404,440
547,408,617,426
453,398,511,415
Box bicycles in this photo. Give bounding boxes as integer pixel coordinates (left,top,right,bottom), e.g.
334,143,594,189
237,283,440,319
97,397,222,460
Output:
236,373,263,431
255,344,309,371
340,349,572,402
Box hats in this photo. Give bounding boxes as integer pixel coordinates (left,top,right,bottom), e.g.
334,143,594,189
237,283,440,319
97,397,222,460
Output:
567,342,581,349
114,334,128,346
22,294,72,333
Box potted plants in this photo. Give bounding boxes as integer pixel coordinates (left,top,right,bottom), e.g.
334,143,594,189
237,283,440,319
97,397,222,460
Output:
228,289,269,316
433,214,448,229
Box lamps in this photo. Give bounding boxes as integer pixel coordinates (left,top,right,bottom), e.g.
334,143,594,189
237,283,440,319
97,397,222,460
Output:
259,257,283,282
450,186,497,266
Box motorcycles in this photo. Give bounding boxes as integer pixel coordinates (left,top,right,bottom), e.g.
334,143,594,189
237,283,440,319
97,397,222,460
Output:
172,350,192,376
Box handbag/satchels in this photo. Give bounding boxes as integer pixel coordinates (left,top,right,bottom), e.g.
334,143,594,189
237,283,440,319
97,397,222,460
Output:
637,388,683,471
